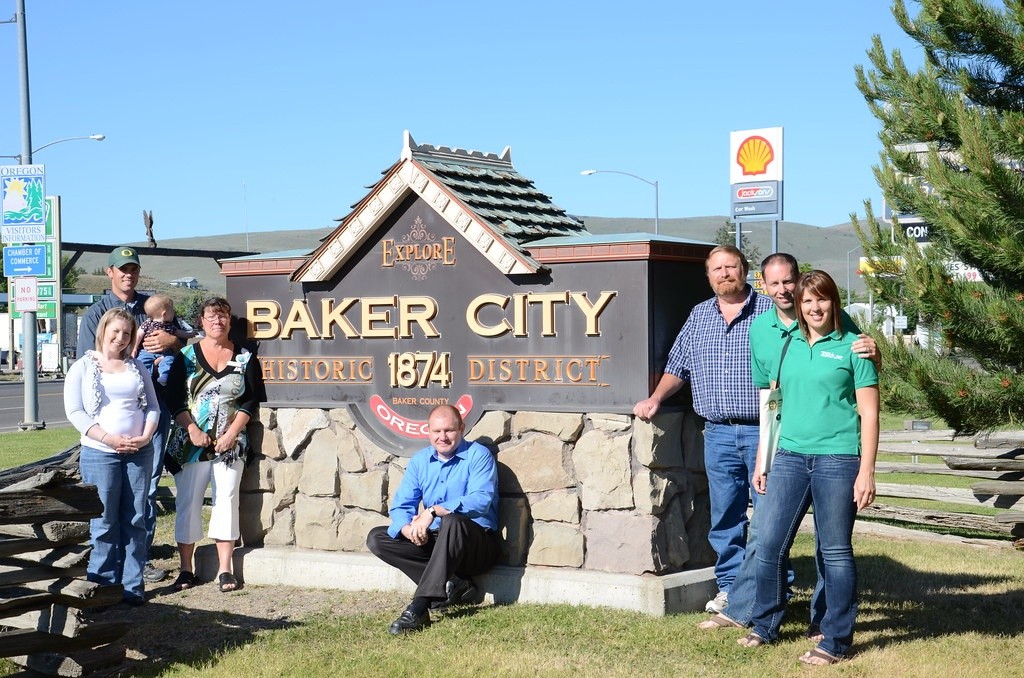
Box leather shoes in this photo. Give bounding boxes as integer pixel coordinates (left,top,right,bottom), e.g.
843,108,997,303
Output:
390,610,432,634
449,580,477,603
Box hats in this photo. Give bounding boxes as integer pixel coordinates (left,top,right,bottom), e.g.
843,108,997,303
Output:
108,246,142,269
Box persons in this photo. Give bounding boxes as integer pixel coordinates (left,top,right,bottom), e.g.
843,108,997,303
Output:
161,298,261,591
63,307,160,612
631,242,792,616
698,245,882,641
77,246,189,583
735,268,880,667
364,405,498,637
129,294,199,394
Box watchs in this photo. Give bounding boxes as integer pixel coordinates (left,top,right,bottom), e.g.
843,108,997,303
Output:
428,506,437,518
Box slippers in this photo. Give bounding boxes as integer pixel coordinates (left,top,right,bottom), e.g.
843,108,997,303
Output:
737,632,763,648
804,625,825,643
800,647,855,669
701,613,745,629
219,572,239,592
173,571,195,591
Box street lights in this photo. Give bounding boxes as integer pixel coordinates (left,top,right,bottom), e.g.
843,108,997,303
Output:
0,1,107,430
580,168,659,235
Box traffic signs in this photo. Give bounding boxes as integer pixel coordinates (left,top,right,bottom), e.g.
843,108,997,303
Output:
3,245,46,277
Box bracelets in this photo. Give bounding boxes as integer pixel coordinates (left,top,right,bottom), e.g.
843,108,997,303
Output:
146,436,151,443
100,433,107,442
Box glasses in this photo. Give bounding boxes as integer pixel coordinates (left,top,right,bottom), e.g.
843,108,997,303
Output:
202,315,231,322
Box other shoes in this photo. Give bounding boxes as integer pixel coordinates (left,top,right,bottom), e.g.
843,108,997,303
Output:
128,596,144,608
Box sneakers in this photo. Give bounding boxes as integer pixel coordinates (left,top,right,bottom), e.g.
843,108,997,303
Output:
705,591,728,613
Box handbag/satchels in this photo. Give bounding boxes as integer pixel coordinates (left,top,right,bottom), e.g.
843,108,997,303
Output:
760,336,795,473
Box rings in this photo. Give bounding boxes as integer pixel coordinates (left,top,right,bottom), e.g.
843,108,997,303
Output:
867,347,870,352
869,498,873,501
